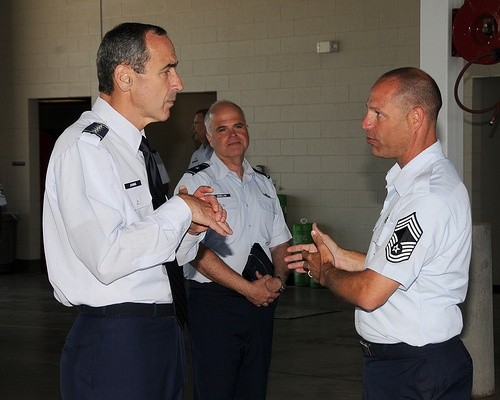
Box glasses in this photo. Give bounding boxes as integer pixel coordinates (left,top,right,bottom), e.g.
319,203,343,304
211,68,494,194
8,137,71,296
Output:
193,121,203,126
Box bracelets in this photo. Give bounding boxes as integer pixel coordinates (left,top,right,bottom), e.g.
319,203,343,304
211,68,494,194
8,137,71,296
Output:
274,274,286,293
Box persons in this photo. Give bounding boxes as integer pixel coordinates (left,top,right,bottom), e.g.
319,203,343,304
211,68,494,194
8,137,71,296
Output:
43,22,234,400
189,107,214,168
172,100,293,400
284,66,474,400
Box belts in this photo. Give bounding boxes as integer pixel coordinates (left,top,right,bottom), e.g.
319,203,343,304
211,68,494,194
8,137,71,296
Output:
78,304,176,318
359,335,459,359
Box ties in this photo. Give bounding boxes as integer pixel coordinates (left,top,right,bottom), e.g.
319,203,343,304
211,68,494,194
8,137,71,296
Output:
139,135,188,330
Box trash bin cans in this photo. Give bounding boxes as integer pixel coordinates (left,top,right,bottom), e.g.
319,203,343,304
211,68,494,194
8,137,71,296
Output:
292,222,323,288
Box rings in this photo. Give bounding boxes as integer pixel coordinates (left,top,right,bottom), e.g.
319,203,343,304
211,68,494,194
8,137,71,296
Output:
307,270,313,279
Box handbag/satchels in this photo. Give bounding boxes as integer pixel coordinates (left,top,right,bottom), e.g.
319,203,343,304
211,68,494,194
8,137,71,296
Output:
242,242,280,314
